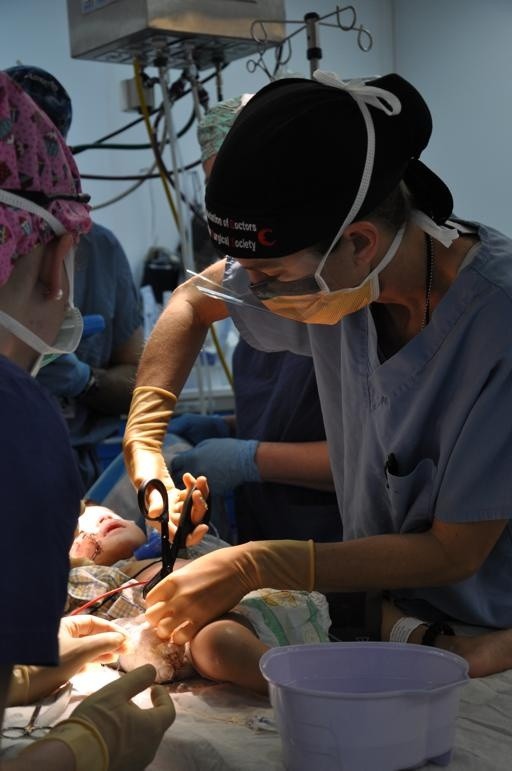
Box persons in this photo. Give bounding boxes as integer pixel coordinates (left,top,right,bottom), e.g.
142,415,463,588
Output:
68,493,511,694
116,71,512,650
164,90,347,545
2,61,150,493
1,71,178,771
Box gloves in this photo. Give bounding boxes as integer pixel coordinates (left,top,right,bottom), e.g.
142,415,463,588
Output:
38,664,178,771
144,540,316,650
169,435,263,504
37,353,92,404
5,614,130,708
170,412,231,447
120,382,185,517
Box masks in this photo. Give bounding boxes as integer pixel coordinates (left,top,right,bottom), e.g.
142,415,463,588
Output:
259,243,379,326
0,189,86,378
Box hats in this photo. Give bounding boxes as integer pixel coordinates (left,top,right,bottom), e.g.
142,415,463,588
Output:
5,62,73,134
0,74,93,290
205,71,435,260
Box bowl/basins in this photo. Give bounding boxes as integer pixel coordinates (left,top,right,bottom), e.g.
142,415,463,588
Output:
260,641,469,769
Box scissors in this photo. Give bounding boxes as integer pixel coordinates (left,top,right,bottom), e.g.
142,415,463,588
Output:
138,479,212,597
